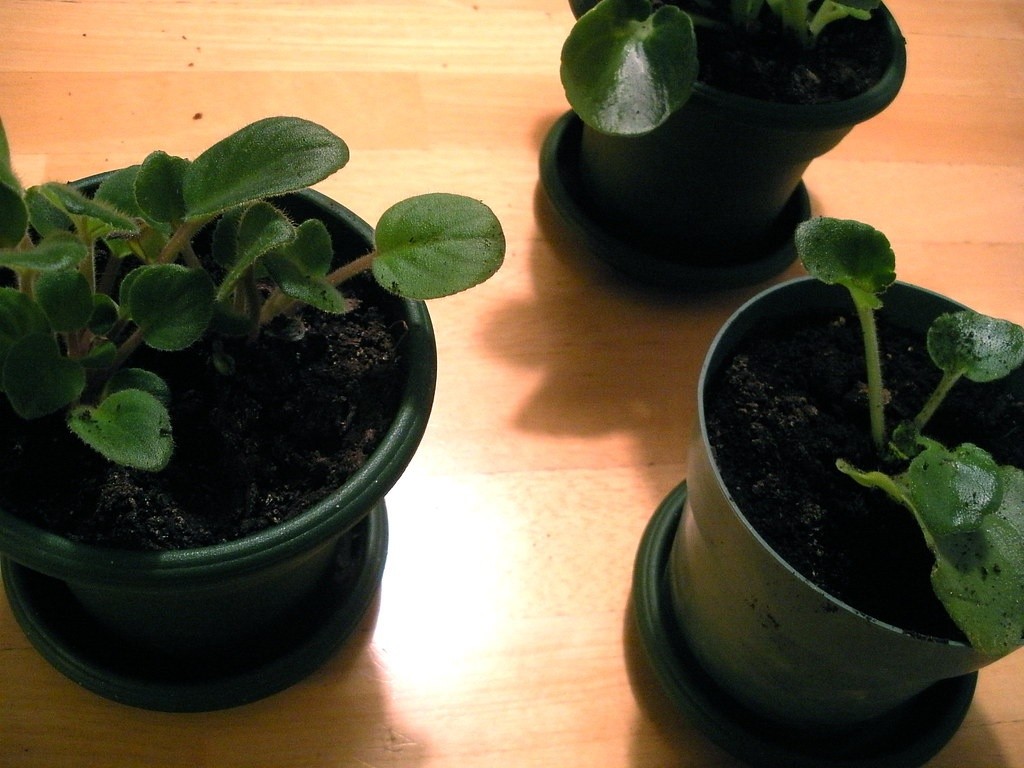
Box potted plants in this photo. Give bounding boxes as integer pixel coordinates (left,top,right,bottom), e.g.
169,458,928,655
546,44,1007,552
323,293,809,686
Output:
0,113,507,714
543,0,907,288
632,219,1024,766
